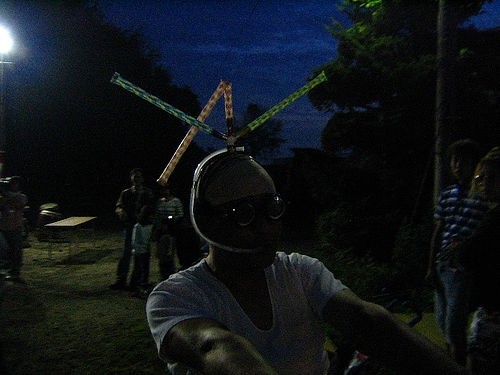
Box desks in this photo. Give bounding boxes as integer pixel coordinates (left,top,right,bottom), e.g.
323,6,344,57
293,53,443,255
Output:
45,217,95,255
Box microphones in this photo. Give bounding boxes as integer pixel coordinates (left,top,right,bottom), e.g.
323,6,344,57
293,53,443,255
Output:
199,229,263,254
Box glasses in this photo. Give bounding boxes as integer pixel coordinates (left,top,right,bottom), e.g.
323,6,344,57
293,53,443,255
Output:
226,194,287,228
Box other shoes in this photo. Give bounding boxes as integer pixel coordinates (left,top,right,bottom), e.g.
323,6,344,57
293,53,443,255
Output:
0,271,149,297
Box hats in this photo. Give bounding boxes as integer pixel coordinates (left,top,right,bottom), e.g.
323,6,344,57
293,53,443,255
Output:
140,205,153,218
189,151,277,253
